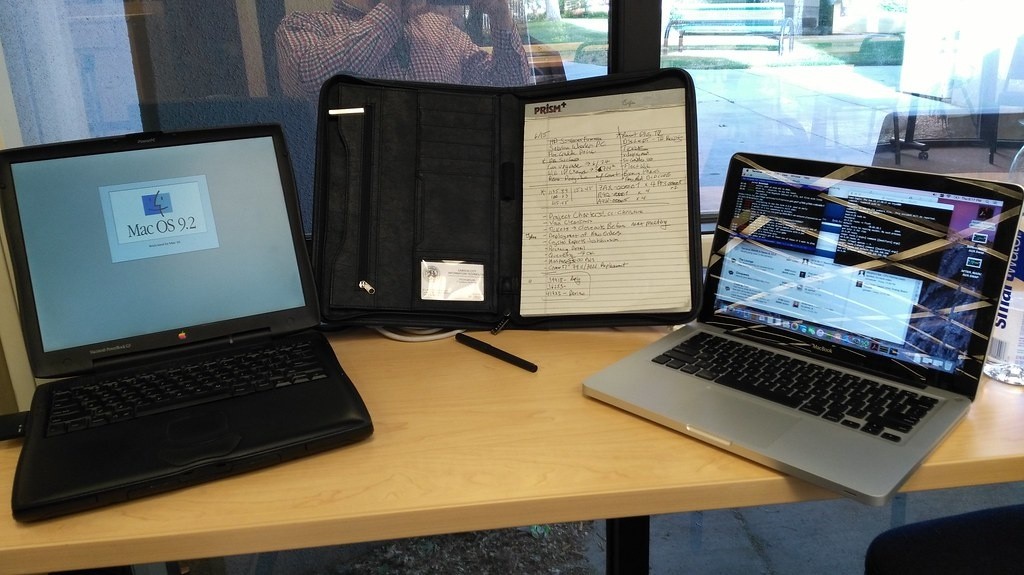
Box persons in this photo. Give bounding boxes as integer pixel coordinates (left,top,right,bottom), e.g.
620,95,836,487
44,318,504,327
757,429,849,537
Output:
275,1,531,98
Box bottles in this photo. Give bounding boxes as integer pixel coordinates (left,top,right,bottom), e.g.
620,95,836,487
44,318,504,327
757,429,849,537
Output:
983,144,1023,389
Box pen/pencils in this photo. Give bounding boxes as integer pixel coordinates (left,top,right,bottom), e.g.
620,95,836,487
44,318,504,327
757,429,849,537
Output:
456,332,538,374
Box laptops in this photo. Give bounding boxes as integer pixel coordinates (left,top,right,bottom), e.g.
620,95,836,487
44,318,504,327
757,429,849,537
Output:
583,153,1024,508
0,123,373,523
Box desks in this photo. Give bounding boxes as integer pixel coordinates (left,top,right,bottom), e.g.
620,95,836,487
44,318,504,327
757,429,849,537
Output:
1,229,1024,574
